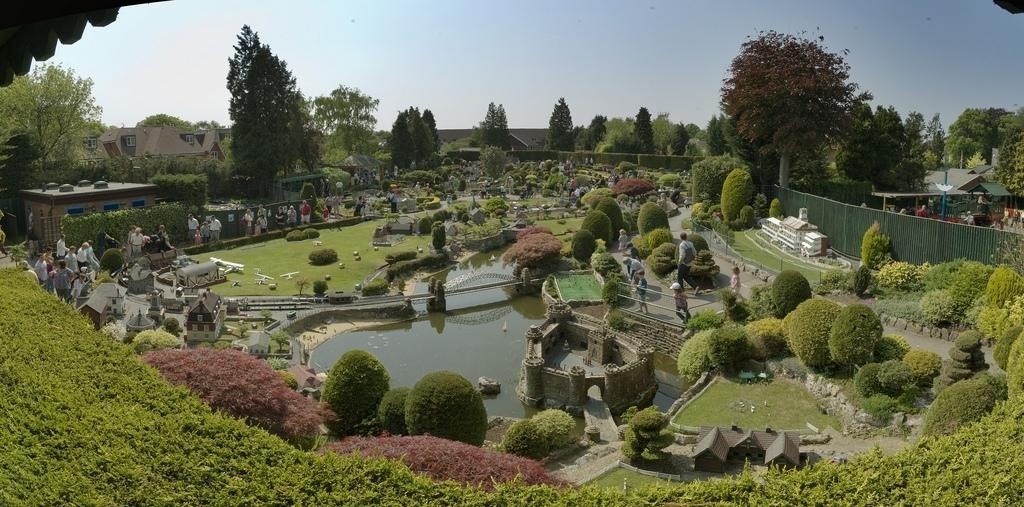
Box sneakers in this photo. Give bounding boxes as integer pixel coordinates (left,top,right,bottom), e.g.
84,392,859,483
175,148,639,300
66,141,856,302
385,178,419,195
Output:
635,308,650,316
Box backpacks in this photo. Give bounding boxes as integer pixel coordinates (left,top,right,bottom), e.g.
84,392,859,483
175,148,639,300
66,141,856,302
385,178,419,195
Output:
682,243,695,264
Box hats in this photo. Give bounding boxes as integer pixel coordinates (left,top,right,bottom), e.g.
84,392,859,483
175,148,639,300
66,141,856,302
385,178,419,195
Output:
669,282,682,291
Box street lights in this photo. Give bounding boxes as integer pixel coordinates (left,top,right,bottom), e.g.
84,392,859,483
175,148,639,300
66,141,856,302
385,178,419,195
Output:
936,171,953,221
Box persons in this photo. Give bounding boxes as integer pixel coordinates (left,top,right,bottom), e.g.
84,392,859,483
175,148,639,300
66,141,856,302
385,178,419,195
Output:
728,266,740,298
963,210,975,225
676,231,699,294
624,241,641,263
188,214,222,246
127,225,143,257
322,168,432,218
242,200,312,236
683,196,689,208
157,224,174,251
669,282,691,322
635,269,647,314
34,229,114,304
915,204,928,217
26,208,36,242
480,155,594,199
617,228,628,252
622,256,643,284
